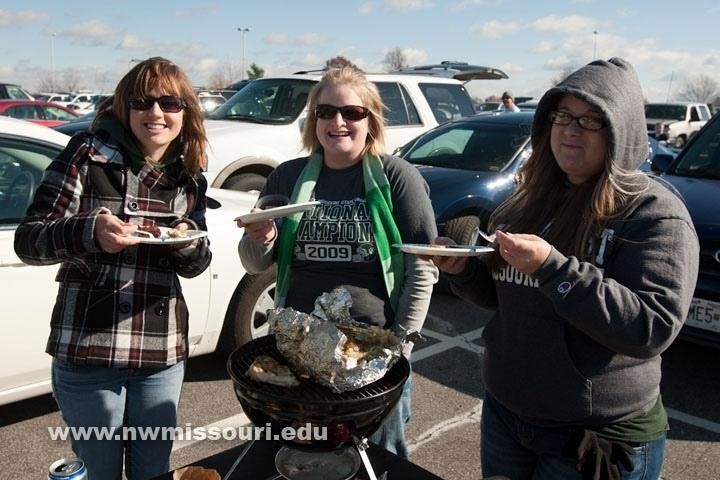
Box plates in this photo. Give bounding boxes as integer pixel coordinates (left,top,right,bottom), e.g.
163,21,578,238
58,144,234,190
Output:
391,243,494,258
233,200,320,224
122,225,207,244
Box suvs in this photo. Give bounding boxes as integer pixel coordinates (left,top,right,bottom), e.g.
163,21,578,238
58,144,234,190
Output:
207,60,509,194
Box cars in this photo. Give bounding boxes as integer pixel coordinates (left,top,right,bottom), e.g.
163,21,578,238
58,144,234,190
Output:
655,113,720,342
389,112,681,249
645,98,712,151
1,116,293,406
1,77,113,123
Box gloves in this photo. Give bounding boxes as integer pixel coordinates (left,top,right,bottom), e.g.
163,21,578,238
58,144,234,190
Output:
570,429,638,479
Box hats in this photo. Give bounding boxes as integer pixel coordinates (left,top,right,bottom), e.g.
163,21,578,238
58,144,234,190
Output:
502,91,514,102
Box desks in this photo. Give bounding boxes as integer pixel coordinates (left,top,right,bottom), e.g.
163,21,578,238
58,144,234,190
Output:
150,441,445,480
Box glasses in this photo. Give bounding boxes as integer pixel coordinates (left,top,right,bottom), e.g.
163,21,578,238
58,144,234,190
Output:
127,95,188,113
502,96,510,99
314,104,370,121
550,109,605,131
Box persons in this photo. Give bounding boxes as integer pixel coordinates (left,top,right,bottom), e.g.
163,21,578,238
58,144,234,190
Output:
502,90,521,112
238,67,440,460
413,57,701,480
648,122,669,141
13,57,212,480
